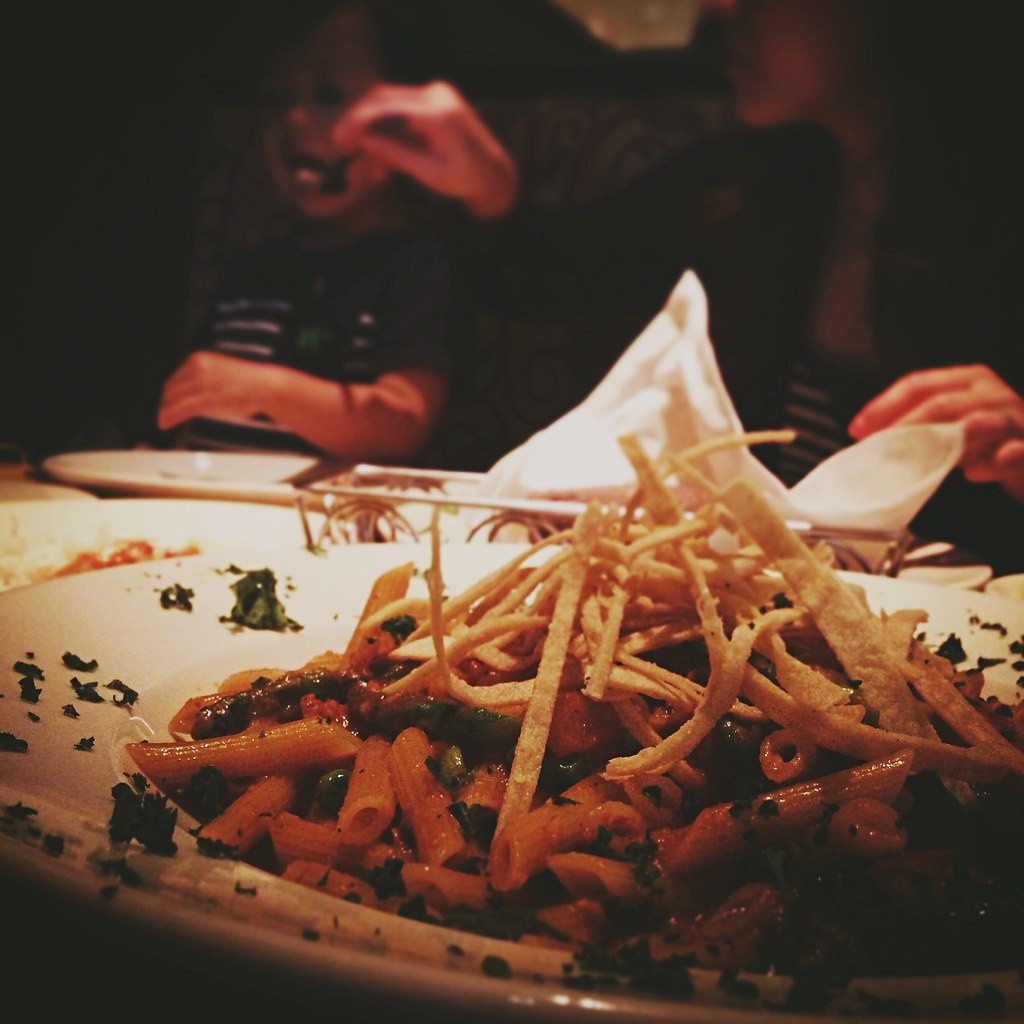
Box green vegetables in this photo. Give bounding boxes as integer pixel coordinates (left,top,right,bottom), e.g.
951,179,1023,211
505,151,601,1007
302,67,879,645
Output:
0,567,1024,1024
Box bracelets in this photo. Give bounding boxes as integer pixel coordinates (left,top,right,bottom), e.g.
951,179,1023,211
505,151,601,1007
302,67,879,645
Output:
336,386,356,416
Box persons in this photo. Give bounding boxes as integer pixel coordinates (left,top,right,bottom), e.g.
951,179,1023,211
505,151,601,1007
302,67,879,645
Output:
324,0,1024,577
157,0,451,465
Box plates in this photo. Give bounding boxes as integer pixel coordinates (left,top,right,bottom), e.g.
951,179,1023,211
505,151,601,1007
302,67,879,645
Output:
0,479,100,505
38,447,494,500
893,541,994,589
981,572,1024,602
499,508,916,580
0,497,395,593
0,541,1024,1024
